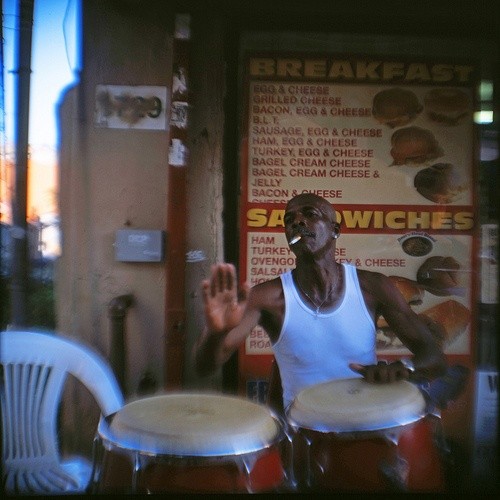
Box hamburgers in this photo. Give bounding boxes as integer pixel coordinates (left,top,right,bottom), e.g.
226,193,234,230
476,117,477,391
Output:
372,87,473,204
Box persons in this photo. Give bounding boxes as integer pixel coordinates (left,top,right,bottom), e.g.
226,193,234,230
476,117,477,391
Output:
192,193,450,414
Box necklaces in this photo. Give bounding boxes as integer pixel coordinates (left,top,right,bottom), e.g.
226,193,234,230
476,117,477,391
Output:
293,264,339,320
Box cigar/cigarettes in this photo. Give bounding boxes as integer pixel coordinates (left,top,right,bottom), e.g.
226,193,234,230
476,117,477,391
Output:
288,236,303,247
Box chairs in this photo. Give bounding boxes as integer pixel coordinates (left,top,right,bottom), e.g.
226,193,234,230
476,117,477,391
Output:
0,331,123,498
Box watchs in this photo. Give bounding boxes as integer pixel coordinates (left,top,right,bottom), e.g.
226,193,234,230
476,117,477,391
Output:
399,357,420,382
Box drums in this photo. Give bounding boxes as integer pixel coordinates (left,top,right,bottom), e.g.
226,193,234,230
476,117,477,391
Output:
98,392,289,494
287,377,444,492
